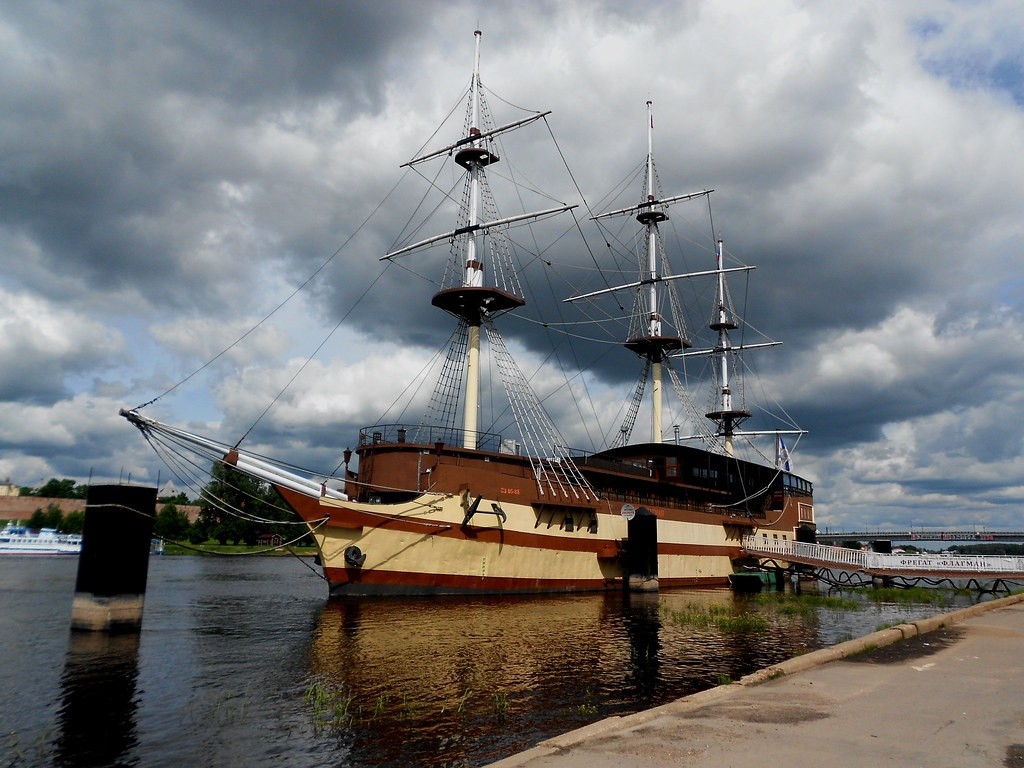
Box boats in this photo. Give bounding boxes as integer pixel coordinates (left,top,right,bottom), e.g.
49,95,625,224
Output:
0,519,82,555
729,570,787,586
784,571,819,582
148,536,166,555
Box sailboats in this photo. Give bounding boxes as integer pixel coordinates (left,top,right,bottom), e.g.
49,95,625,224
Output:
120,29,817,603
